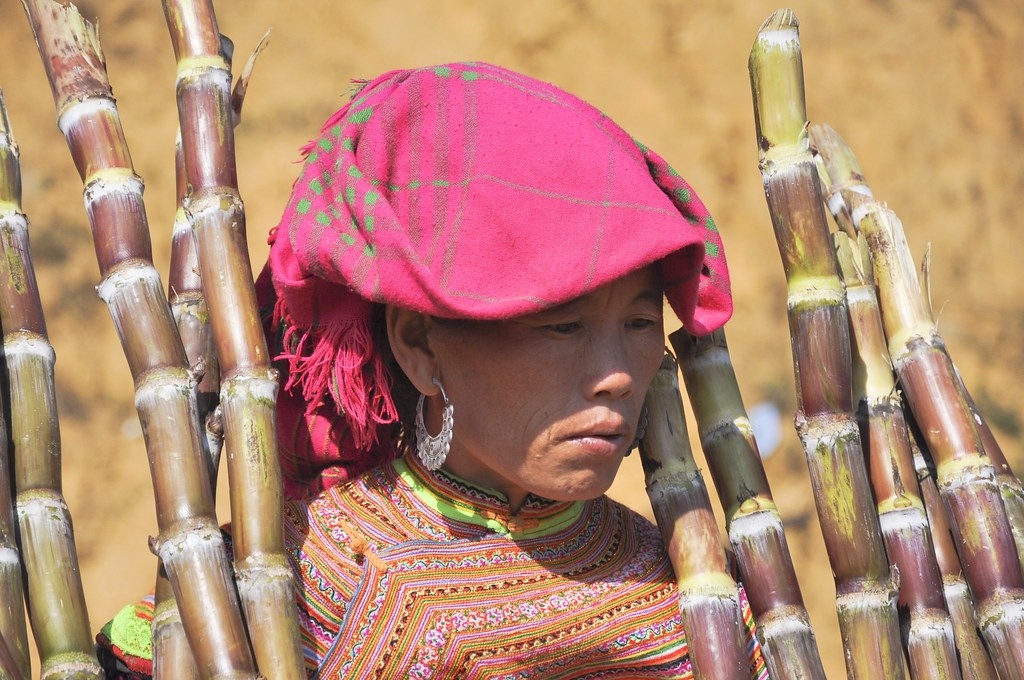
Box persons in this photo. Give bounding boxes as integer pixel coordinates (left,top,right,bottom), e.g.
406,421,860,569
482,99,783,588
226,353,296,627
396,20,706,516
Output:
94,61,775,680
